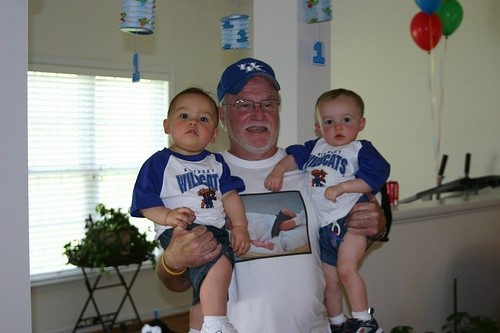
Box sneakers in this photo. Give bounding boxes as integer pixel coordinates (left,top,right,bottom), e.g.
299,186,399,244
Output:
330,315,349,333
200,322,238,333
343,307,383,333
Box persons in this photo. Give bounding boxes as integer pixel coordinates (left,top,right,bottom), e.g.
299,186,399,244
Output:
155,58,387,333
129,87,251,333
264,88,391,333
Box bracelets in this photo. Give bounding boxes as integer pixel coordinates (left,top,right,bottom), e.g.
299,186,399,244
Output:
162,249,187,275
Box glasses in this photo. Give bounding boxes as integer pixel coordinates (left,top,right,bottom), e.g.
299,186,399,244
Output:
222,99,280,112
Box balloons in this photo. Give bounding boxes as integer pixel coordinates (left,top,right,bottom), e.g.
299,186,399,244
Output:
410,0,464,55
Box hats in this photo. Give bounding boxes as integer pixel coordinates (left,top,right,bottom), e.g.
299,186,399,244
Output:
217,58,281,103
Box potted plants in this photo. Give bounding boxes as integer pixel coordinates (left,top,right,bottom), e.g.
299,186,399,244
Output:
63,203,159,279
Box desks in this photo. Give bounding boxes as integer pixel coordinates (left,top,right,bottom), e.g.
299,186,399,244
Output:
69,259,148,333
